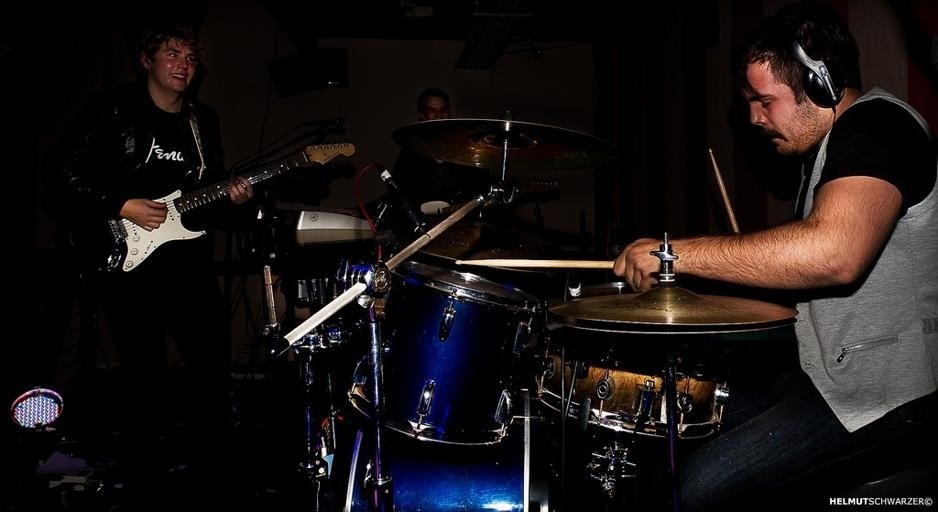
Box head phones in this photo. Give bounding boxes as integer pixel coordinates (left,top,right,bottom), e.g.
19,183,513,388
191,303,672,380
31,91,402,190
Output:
763,15,845,107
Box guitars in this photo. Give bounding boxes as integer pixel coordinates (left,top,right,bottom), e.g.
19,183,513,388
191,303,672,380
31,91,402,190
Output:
94,139,356,274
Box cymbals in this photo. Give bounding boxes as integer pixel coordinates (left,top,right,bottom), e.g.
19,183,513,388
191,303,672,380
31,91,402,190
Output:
418,241,572,274
549,287,798,336
392,119,594,167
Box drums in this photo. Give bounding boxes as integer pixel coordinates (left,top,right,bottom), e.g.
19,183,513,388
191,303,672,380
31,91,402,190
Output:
345,430,550,512
349,255,542,445
548,349,715,430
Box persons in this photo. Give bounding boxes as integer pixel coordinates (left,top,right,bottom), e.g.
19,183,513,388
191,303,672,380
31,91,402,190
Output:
389,88,492,250
43,25,256,504
610,0,937,509
